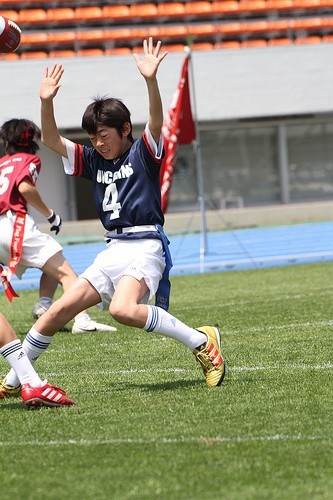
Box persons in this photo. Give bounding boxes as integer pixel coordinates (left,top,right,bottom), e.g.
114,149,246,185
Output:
0,118,119,335
0,36,225,399
0,312,74,409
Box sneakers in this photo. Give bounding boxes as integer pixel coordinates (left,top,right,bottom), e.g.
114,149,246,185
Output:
72,318,117,334
32,302,70,332
21,379,75,409
0,378,22,399
193,326,225,388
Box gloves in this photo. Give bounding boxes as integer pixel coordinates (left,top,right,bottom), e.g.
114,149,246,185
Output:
47,209,62,235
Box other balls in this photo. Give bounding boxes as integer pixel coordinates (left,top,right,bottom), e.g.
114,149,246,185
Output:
0,15,23,53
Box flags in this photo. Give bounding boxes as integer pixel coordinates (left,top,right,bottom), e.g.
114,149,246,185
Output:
159,56,197,215
